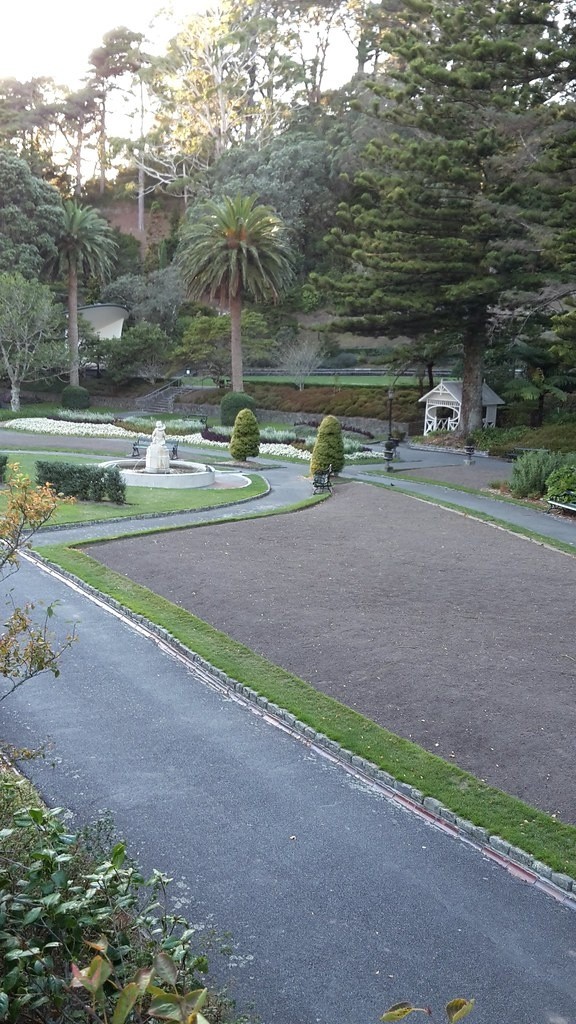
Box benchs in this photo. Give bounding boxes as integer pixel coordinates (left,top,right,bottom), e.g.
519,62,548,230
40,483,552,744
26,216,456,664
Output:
131,439,179,460
546,490,576,515
313,464,333,494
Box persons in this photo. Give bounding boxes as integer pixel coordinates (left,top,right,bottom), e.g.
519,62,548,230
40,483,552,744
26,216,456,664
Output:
151,421,166,445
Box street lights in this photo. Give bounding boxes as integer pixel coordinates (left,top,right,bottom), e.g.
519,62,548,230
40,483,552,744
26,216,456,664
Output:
387,386,394,442
